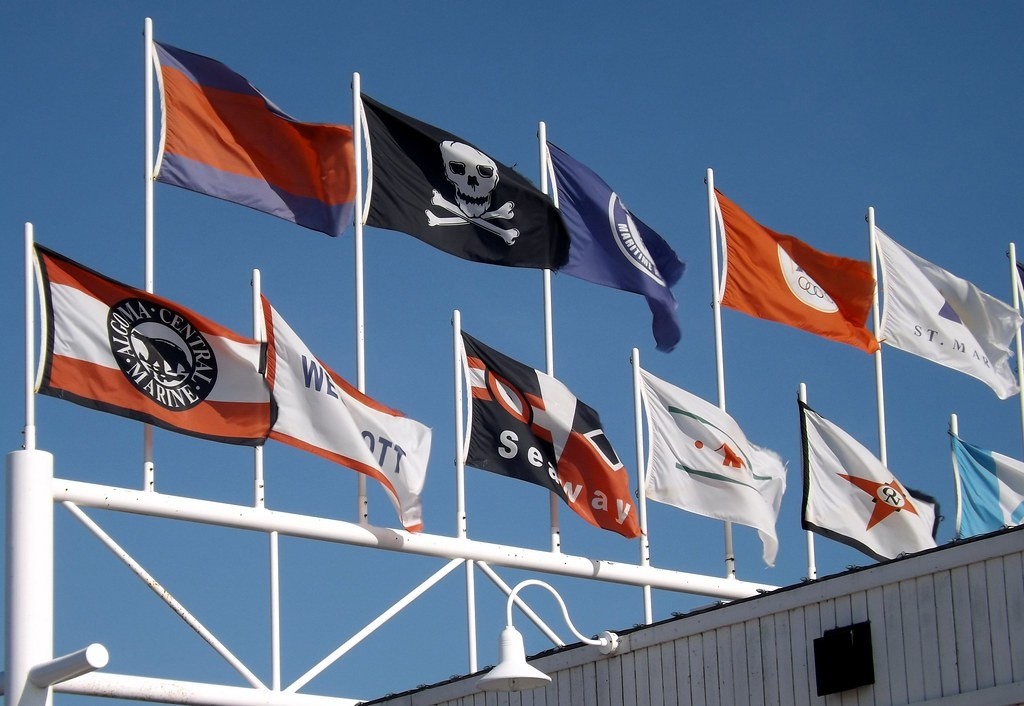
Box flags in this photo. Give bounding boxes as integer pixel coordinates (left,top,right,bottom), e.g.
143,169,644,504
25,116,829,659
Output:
874,224,1024,400
947,429,1024,540
33,241,278,448
261,292,432,535
639,366,790,570
460,329,645,540
360,91,571,275
152,40,356,238
546,140,687,354
797,393,945,563
714,187,882,355
